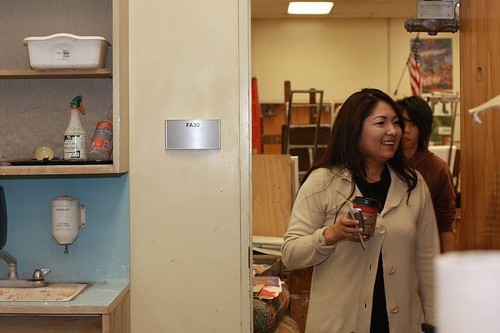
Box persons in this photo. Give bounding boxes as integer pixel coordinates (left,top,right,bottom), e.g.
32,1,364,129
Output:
281,89,440,333
396,97,455,231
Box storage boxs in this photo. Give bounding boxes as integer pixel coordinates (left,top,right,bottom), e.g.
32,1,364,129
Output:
22,33,112,70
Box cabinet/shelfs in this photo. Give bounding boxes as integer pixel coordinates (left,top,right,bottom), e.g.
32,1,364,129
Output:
0,0,130,177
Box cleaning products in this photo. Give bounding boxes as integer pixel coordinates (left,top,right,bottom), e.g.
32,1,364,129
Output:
85,105,114,160
61,94,88,159
50,196,81,245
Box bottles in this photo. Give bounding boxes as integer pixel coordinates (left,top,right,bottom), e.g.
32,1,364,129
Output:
87,104,114,161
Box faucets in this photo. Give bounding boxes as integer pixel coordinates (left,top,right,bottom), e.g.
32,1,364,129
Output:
1,250,19,281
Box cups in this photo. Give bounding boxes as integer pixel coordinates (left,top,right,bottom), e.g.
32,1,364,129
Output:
348,195,378,235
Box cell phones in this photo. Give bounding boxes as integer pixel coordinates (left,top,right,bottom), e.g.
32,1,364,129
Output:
348,208,365,236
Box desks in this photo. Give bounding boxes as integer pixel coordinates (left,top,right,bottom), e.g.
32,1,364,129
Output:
0,284,131,333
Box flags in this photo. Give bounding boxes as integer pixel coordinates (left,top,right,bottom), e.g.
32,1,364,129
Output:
406,38,422,98
